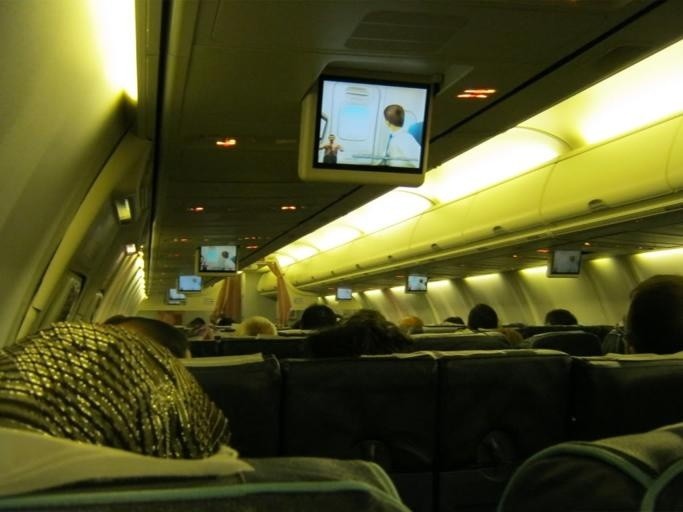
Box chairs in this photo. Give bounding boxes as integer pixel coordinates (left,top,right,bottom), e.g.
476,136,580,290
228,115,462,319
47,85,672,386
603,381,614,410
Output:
0,317,682,512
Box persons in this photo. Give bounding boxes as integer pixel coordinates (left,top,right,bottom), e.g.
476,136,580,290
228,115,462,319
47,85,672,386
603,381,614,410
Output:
318,134,344,164
222,252,236,272
384,105,421,168
566,256,579,273
102,274,682,359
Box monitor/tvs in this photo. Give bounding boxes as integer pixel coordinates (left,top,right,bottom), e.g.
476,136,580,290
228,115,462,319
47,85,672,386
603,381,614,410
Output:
167,274,203,305
548,248,583,278
298,67,433,187
194,245,239,276
336,287,352,300
405,274,428,293
